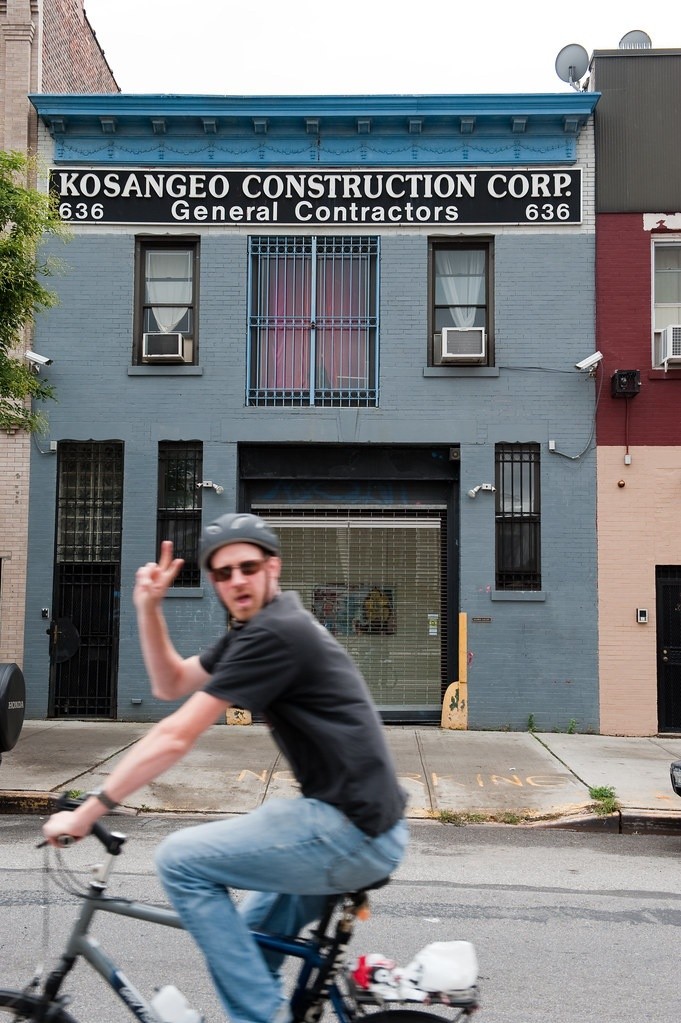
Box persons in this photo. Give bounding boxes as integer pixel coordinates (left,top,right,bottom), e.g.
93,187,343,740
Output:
37,513,409,1022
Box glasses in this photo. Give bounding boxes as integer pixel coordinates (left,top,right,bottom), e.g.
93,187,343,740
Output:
209,559,268,582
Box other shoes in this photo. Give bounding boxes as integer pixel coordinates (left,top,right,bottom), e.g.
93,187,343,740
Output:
275,1000,294,1023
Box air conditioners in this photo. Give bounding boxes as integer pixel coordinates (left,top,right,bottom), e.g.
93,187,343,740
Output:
142,332,185,363
659,324,681,373
441,326,485,364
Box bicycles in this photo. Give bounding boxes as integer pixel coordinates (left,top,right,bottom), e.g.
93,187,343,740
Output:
1,789,485,1023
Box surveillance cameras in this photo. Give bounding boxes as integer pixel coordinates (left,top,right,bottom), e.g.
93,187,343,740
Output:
576,351,604,371
24,351,53,367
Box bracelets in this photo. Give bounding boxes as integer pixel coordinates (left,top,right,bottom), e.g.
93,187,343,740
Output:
93,788,119,810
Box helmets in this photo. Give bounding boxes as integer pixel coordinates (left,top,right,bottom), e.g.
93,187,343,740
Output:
195,512,282,570
